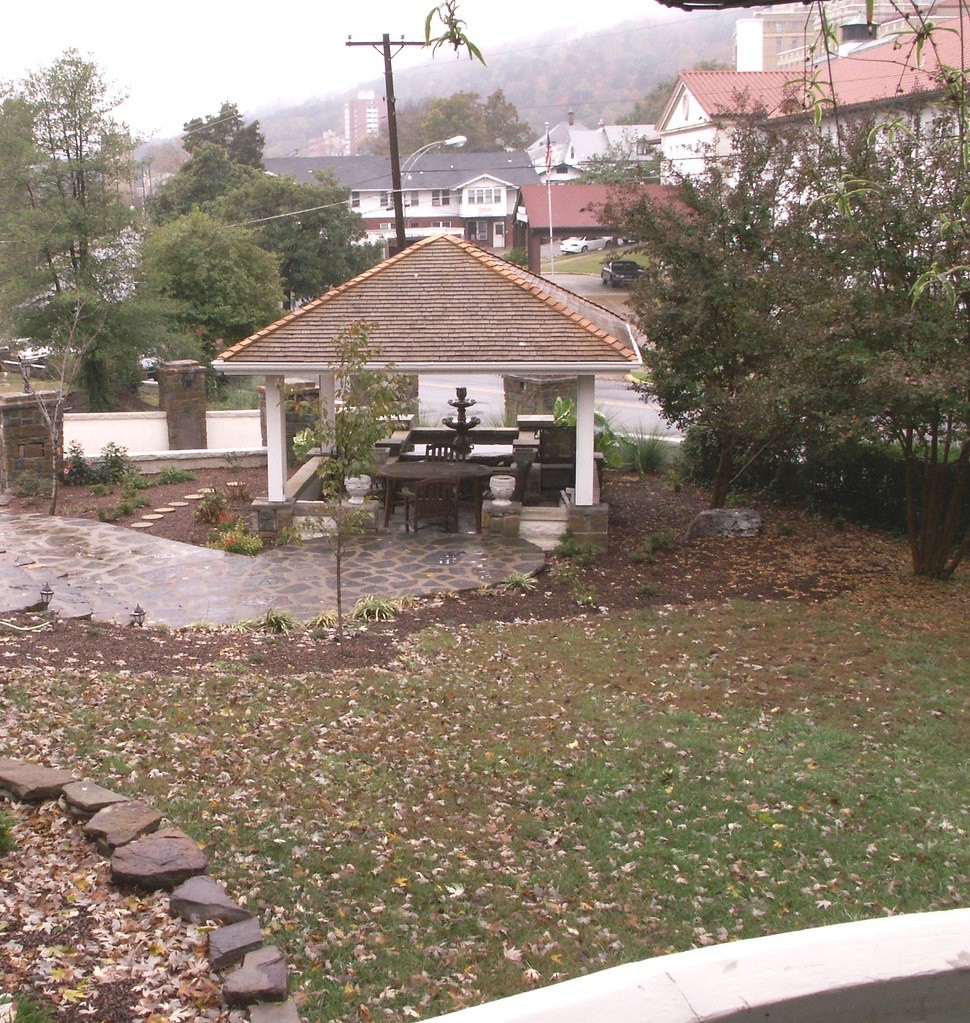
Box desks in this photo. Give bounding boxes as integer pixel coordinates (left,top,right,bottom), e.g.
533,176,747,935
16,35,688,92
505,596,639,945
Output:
380,461,492,535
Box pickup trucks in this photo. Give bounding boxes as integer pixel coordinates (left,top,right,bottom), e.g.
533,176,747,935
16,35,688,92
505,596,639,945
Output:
601,261,645,288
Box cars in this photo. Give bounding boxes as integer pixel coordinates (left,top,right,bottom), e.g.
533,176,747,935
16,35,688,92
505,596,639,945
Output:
559,235,614,255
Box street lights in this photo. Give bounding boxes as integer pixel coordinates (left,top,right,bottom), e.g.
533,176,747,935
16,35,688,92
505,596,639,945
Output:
393,135,467,251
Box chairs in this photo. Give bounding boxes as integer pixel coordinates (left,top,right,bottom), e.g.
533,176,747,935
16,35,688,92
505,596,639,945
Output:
425,442,464,500
481,460,533,503
406,476,459,535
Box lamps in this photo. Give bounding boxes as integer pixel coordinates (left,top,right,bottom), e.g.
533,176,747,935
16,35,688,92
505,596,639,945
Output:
132,604,146,628
20,359,32,394
40,581,54,605
